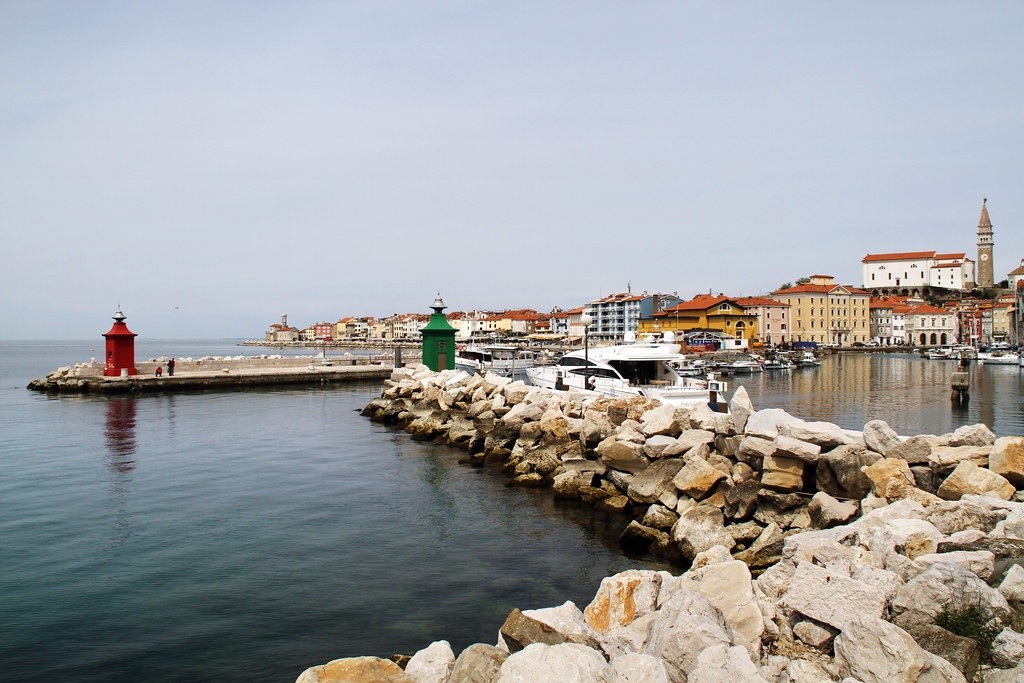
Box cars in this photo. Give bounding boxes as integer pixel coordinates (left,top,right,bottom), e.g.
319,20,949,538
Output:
826,341,840,348
867,341,879,347
854,341,864,347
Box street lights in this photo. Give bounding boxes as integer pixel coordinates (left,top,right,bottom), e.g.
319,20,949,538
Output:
579,313,594,390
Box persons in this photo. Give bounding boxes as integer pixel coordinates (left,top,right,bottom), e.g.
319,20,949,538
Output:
167,358,176,376
156,366,162,377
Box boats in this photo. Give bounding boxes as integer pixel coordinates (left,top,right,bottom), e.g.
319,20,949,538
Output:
925,343,1024,367
454,345,536,375
673,345,824,377
524,343,730,414
